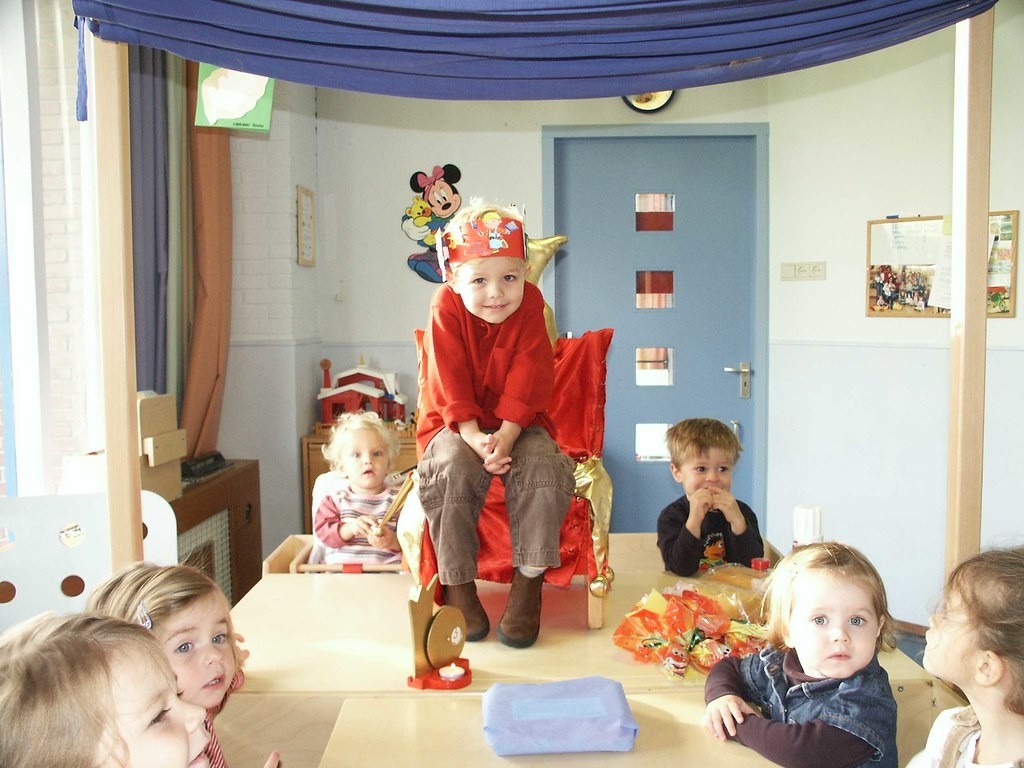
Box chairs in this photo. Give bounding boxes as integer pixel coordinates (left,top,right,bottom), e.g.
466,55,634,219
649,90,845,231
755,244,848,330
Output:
413,327,615,629
258,470,411,573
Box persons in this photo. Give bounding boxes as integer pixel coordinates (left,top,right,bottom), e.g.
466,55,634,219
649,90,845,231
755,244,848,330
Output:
874,265,930,311
0,612,210,768
657,418,764,577
903,545,1024,768
416,206,575,649
87,562,281,768
705,542,898,768
311,412,409,575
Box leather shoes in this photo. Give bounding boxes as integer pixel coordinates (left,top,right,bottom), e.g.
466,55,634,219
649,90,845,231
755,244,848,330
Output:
495,566,545,647
443,580,490,642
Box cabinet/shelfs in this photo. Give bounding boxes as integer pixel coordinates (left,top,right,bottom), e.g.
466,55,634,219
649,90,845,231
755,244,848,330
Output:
302,428,417,533
168,459,263,613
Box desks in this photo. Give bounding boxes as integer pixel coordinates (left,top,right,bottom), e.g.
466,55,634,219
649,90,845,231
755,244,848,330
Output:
227,574,783,768
